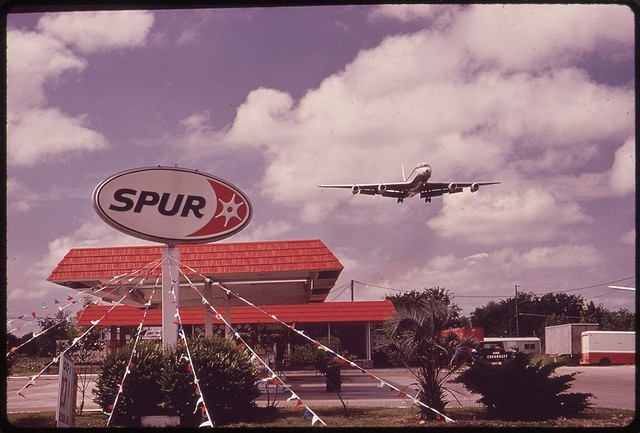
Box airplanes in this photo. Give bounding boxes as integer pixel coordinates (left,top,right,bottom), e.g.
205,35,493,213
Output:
317,160,501,204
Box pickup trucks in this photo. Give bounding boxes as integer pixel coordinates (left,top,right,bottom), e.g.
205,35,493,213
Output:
469,340,513,366
